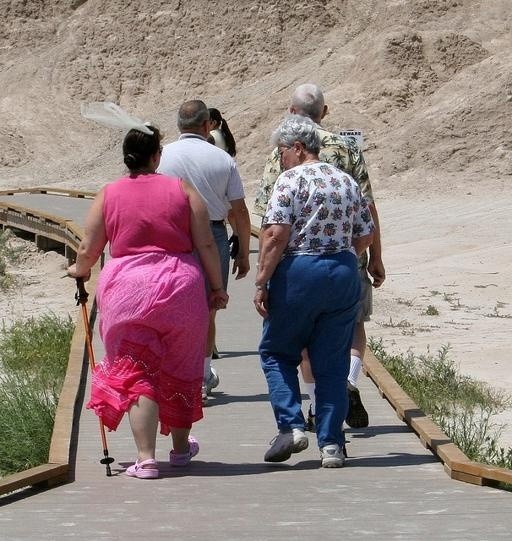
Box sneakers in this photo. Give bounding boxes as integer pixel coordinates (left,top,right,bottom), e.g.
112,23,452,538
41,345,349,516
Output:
345,379,369,428
201,366,219,405
264,428,308,462
308,403,317,431
319,442,346,468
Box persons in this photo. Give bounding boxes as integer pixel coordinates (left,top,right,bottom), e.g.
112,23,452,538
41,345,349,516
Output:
251,83,385,468
67,100,251,479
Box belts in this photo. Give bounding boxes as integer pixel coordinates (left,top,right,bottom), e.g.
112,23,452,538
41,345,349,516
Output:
210,219,225,226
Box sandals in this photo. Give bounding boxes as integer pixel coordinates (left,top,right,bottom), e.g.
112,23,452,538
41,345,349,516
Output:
169,435,200,466
125,459,158,479
